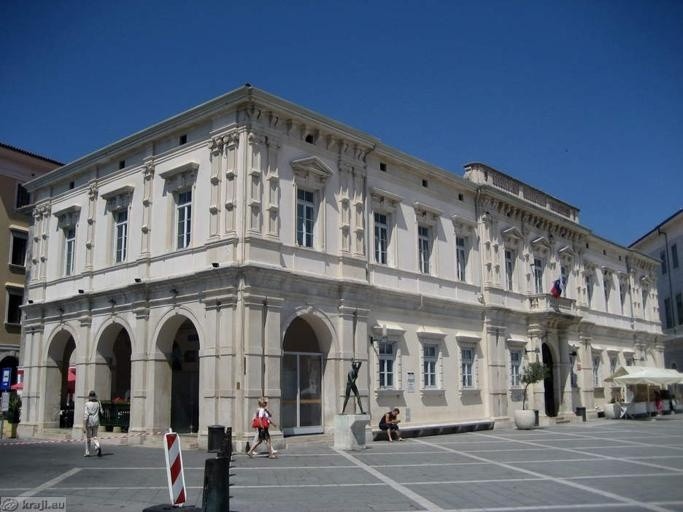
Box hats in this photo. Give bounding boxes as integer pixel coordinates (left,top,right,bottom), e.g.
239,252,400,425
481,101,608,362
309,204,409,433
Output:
87,391,98,399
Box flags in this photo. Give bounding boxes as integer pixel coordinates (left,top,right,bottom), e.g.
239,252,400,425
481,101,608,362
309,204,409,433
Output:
551,279,562,300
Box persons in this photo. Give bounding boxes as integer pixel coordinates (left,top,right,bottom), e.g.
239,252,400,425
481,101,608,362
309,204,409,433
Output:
83,391,104,457
341,361,367,416
8,390,22,438
247,397,279,459
379,408,402,441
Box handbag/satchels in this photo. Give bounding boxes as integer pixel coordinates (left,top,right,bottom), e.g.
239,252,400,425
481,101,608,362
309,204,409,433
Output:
251,412,269,428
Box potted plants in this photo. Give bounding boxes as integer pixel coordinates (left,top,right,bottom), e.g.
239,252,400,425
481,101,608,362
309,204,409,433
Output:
3,394,22,439
514,361,551,429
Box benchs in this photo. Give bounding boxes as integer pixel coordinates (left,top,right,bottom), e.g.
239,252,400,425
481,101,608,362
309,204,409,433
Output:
368,419,495,441
